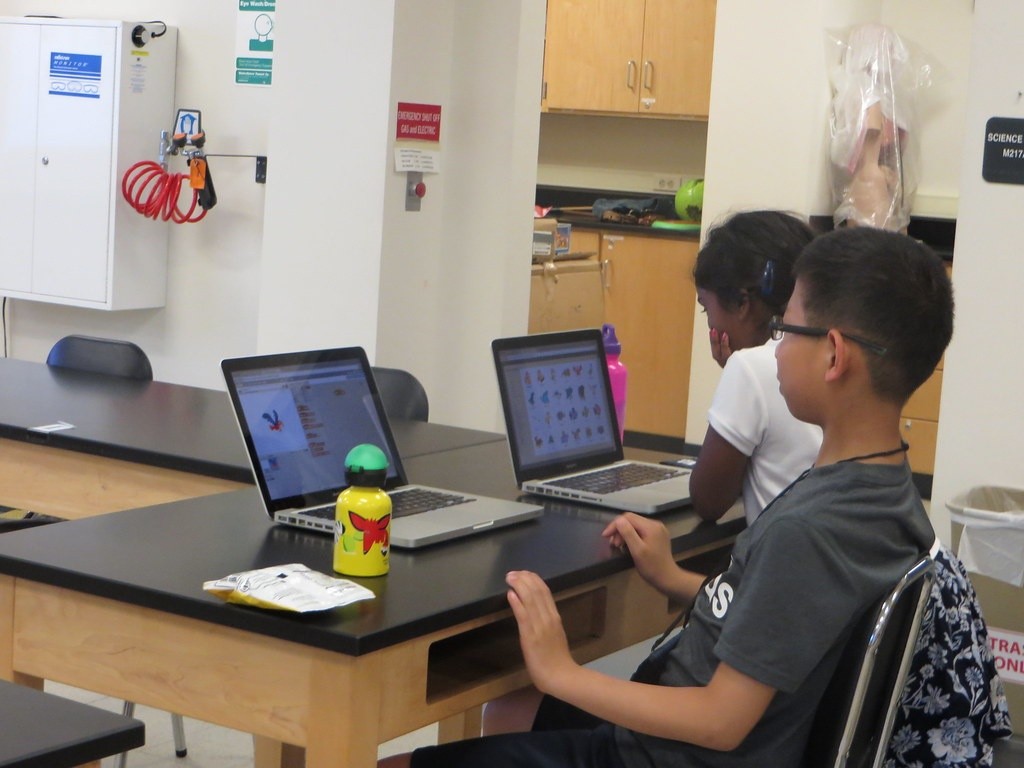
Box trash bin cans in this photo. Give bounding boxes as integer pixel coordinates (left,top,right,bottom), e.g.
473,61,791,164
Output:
948,484,1024,746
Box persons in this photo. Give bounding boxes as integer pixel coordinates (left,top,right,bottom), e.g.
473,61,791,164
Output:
373,229,956,768
688,210,824,534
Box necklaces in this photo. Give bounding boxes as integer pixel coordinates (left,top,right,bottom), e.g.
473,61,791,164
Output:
755,437,912,522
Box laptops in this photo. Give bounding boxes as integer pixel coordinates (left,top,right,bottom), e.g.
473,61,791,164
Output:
493,328,695,516
220,346,547,549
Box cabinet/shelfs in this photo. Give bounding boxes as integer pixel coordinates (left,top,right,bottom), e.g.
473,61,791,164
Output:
539,1,716,124
544,213,700,451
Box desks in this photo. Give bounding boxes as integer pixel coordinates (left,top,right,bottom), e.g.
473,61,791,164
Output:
1,358,748,768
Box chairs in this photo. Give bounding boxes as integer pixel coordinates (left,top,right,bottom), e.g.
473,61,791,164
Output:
1,678,145,768
802,552,940,768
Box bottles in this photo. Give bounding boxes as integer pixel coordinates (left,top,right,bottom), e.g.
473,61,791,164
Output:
601,324,627,446
333,444,391,577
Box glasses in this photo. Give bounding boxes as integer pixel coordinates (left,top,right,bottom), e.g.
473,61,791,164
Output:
768,315,888,356
